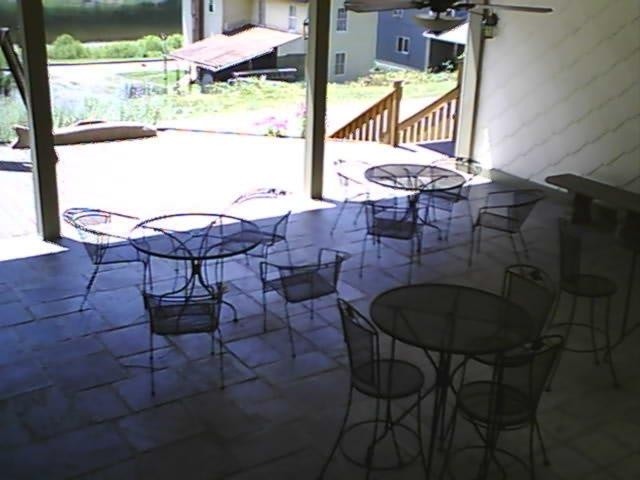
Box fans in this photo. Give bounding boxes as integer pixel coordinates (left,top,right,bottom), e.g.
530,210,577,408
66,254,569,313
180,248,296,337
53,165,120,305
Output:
345,1,553,17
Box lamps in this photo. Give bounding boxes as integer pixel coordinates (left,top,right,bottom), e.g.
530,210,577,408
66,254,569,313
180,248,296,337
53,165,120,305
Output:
476,12,501,40
414,15,467,34
